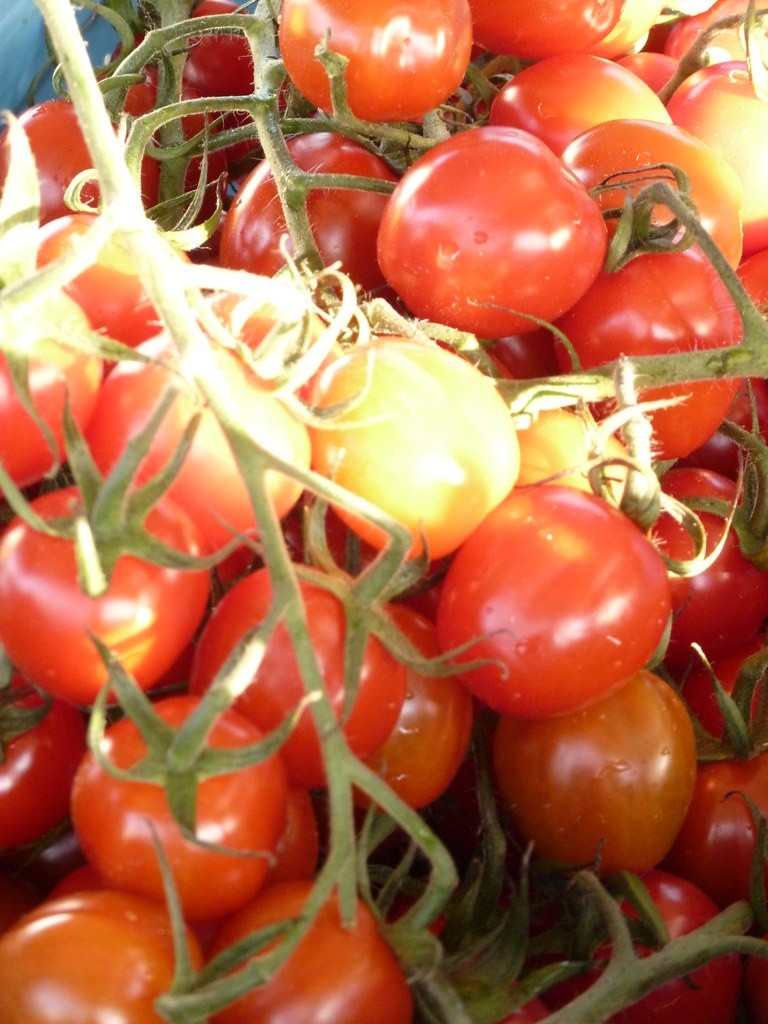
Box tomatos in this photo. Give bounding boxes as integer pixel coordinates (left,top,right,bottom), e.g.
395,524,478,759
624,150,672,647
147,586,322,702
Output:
0,0,768,1024
